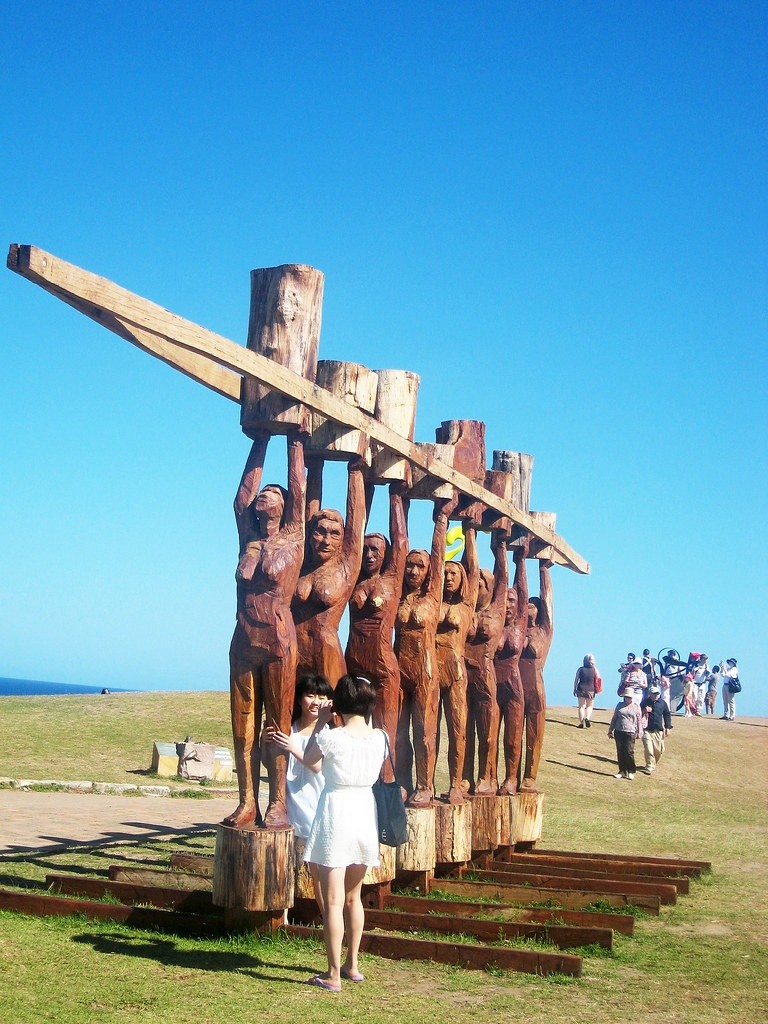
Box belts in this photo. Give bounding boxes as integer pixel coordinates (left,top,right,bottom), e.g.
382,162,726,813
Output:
724,683,729,685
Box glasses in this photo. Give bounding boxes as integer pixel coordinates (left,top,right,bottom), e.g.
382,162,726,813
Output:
627,657,634,660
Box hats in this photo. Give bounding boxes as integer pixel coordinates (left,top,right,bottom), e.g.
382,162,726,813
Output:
619,687,634,698
649,687,660,694
685,674,693,682
727,658,737,667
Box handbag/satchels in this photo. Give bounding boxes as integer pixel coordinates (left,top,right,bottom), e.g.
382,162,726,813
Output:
594,668,601,693
617,671,631,695
728,674,741,693
373,729,410,847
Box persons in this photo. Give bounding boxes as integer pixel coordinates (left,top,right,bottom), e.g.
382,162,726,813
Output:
222,397,312,830
705,665,720,714
490,526,529,796
574,653,598,729
638,687,673,775
512,546,555,793
289,438,372,728
344,468,409,808
260,675,390,994
720,658,738,720
618,650,709,718
460,507,507,796
608,686,644,780
394,489,460,808
428,489,481,806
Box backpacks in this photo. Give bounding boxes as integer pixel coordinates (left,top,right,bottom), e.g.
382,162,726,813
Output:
686,652,708,676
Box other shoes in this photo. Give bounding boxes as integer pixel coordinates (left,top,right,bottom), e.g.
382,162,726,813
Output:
585,719,590,727
728,717,734,721
628,773,634,780
614,771,627,779
644,768,652,774
695,712,701,716
719,716,729,720
577,724,583,728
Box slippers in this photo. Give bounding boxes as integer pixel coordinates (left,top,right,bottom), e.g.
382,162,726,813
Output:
339,966,364,982
305,972,342,992
